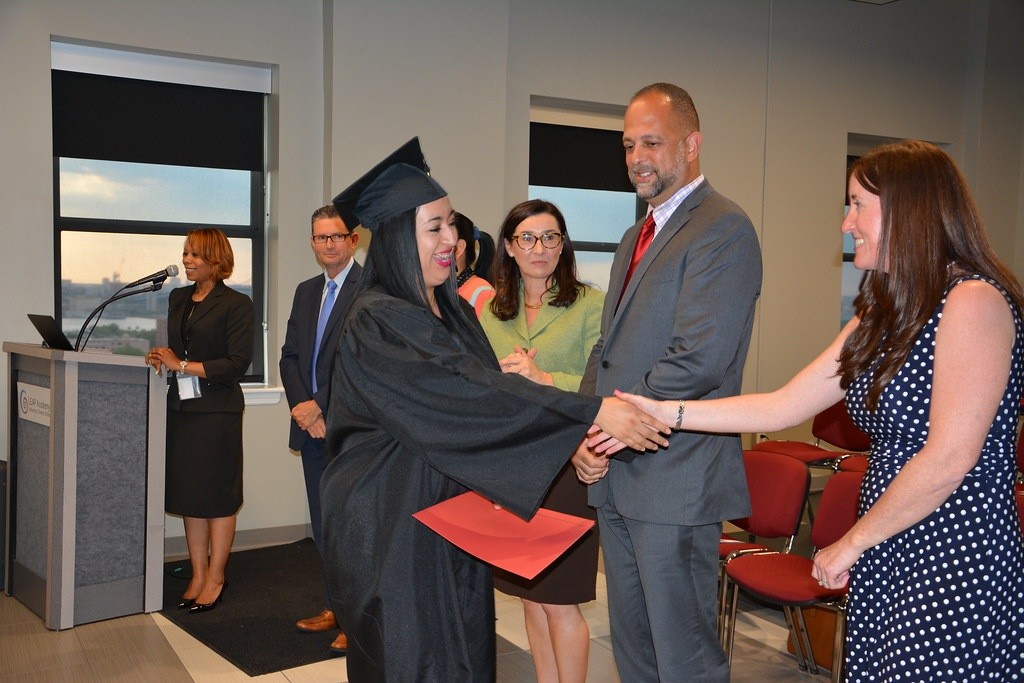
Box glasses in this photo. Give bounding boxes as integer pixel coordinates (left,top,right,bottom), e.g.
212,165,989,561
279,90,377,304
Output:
511,232,563,251
312,233,352,242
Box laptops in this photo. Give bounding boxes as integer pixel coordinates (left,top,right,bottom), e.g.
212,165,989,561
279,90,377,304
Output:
27,314,74,351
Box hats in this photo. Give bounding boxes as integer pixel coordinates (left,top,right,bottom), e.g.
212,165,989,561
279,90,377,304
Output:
332,133,448,228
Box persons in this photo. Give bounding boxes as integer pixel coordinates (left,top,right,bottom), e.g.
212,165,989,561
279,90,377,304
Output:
588,139,1024,683
146,227,255,614
571,82,762,683
480,200,607,683
279,205,368,652
319,136,671,683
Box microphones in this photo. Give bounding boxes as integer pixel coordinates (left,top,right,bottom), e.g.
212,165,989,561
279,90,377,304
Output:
129,265,179,287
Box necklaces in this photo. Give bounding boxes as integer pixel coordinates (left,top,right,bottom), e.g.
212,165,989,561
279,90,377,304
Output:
526,304,542,309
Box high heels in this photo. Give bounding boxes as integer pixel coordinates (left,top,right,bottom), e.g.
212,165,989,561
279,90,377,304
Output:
175,575,229,612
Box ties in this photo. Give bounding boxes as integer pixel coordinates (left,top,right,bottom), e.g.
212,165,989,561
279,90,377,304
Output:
312,279,338,395
618,210,656,306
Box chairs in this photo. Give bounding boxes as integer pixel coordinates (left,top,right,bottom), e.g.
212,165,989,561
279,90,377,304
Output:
713,398,1024,683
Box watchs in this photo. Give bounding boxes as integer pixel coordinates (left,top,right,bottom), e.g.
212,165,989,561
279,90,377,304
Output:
179,360,188,375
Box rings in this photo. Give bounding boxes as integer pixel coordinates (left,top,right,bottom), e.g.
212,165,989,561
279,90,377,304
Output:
818,580,827,586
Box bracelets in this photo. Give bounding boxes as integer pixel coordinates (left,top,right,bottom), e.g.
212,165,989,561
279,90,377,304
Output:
674,399,686,431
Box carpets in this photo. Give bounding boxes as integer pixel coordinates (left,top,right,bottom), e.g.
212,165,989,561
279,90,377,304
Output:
158,538,347,677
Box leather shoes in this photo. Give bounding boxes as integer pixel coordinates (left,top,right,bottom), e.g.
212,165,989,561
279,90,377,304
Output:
296,609,343,630
329,631,349,651
